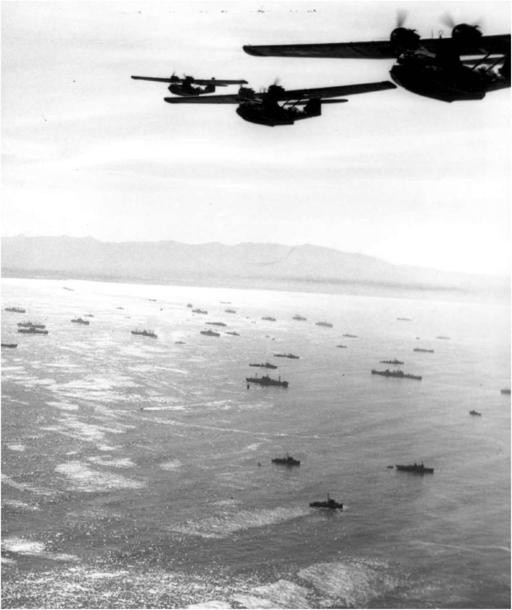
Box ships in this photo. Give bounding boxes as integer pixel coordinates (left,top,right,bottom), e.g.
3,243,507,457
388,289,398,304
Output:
272,453,300,466
397,459,434,474
309,492,343,510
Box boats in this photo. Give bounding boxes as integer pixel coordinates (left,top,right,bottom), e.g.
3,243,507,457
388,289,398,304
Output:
248,361,282,369
274,351,300,361
244,374,291,391
2,343,19,349
6,306,26,314
71,318,90,325
373,369,422,382
133,330,158,338
200,329,221,337
15,321,50,335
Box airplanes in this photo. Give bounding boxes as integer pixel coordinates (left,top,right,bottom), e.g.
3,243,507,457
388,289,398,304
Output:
242,7,512,104
163,76,399,128
130,71,248,97
